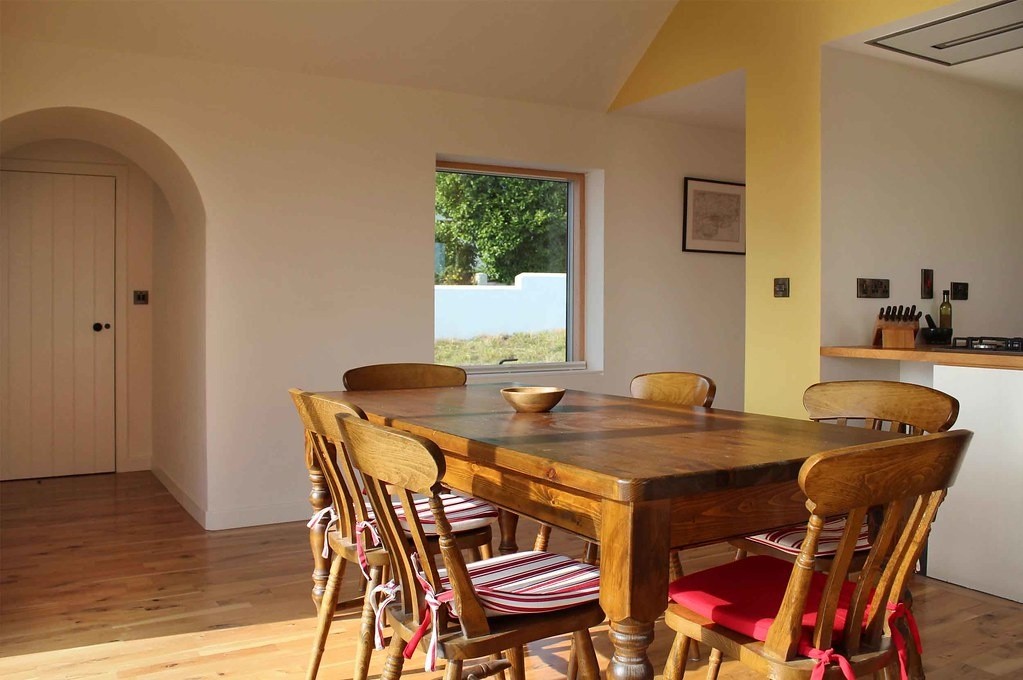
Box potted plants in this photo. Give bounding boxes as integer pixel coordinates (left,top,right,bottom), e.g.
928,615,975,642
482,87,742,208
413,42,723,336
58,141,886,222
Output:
471,264,499,285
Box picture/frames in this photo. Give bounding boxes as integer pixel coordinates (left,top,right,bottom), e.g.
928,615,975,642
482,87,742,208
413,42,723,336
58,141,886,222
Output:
681,177,746,254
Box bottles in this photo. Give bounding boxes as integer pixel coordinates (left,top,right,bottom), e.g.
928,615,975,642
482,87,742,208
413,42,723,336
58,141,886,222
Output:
940,290,952,328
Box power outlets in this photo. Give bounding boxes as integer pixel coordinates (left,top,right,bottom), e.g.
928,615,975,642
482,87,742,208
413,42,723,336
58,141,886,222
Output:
951,282,969,300
857,278,889,298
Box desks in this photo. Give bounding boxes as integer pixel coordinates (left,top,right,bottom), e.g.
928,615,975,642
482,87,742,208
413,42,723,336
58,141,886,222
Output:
315,382,911,680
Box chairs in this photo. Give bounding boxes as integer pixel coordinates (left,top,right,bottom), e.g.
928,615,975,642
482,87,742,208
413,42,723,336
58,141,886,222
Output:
288,386,520,680
706,380,961,680
567,371,717,680
661,430,973,680
336,413,605,680
342,363,493,680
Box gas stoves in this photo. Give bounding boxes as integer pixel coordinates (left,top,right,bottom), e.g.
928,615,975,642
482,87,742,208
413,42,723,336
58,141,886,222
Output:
931,337,1023,357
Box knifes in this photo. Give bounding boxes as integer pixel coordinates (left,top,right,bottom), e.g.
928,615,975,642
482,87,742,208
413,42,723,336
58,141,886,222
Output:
871,305,922,348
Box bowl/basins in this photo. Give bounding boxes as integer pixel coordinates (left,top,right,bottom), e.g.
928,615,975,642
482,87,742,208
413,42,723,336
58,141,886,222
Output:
499,386,565,413
921,328,952,346
